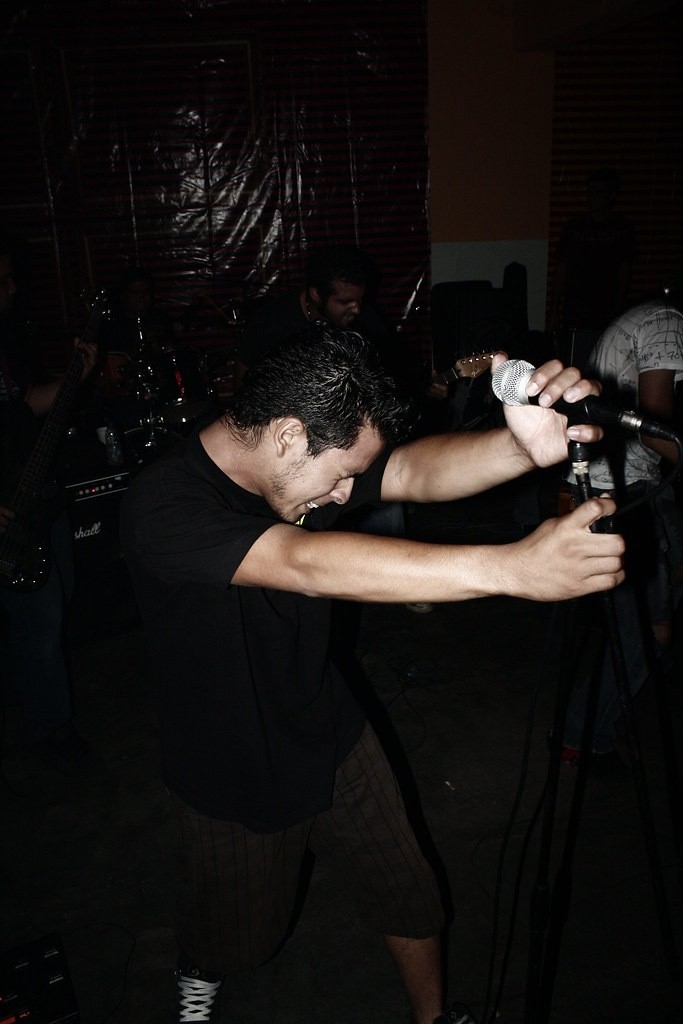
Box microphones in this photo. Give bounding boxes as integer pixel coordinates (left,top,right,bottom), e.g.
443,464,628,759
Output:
490,359,674,439
396,274,426,335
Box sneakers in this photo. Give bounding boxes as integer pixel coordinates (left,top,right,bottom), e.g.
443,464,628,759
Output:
174,954,225,1024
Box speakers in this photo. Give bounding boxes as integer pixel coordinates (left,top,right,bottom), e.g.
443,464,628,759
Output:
50,469,131,595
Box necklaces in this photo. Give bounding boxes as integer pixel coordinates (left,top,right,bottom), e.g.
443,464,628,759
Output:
306,305,327,325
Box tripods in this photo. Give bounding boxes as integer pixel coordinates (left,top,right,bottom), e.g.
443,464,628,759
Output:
524,441,683,1024
121,312,183,455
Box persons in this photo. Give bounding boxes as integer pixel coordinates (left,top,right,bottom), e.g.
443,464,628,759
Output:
101,269,174,361
231,252,448,614
559,298,683,779
119,325,626,1024
0,247,101,788
